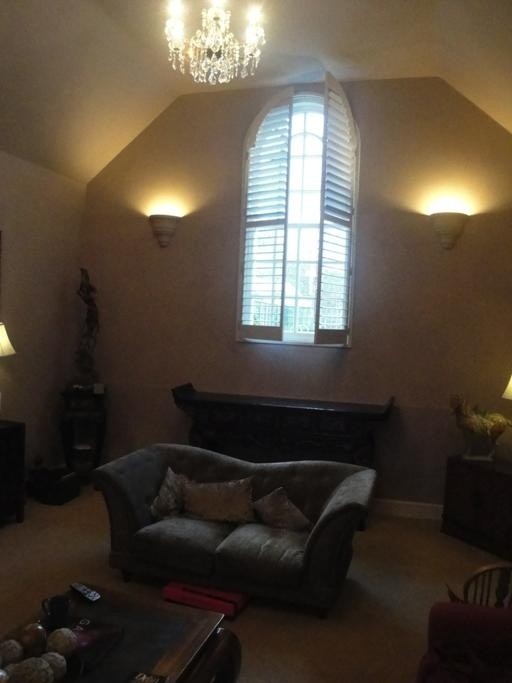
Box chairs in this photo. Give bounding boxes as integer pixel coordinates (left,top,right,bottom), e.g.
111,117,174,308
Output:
463,563,512,607
415,603,512,683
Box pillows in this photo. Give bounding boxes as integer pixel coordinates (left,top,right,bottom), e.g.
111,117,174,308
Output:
249,487,311,532
150,465,185,520
179,474,258,522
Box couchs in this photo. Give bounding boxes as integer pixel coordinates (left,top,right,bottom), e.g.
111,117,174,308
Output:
91,443,380,620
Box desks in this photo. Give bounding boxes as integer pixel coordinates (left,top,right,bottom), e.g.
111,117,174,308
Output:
170,382,395,464
0,585,241,683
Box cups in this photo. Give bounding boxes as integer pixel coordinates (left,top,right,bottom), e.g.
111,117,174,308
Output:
41,596,72,620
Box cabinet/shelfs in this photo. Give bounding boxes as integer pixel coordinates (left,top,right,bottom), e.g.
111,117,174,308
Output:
60,382,108,485
441,445,512,561
0,418,26,523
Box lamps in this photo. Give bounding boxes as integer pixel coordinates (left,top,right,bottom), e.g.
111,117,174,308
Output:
149,215,182,247
501,374,512,400
423,212,471,249
0,321,17,357
164,0,267,85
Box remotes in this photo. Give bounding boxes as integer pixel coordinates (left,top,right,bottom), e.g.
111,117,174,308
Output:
70,581,101,602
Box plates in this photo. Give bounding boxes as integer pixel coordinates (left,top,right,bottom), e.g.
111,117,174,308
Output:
42,613,76,630
